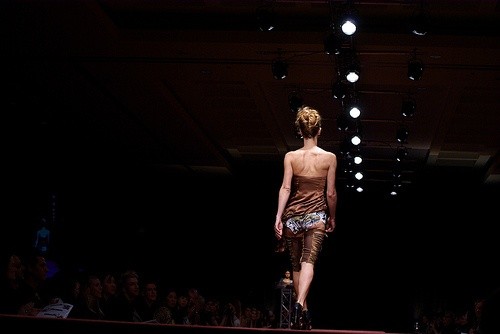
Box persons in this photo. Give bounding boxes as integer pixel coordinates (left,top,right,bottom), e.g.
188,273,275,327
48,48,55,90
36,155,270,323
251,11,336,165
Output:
274,106,338,328
277,271,294,327
403,288,499,334
3,253,276,328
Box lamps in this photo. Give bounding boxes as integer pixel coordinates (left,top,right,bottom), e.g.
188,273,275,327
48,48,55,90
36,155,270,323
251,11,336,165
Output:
325,1,434,201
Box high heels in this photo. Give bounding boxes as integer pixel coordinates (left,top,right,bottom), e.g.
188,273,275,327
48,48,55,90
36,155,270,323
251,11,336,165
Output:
302,306,312,330
291,303,304,329
